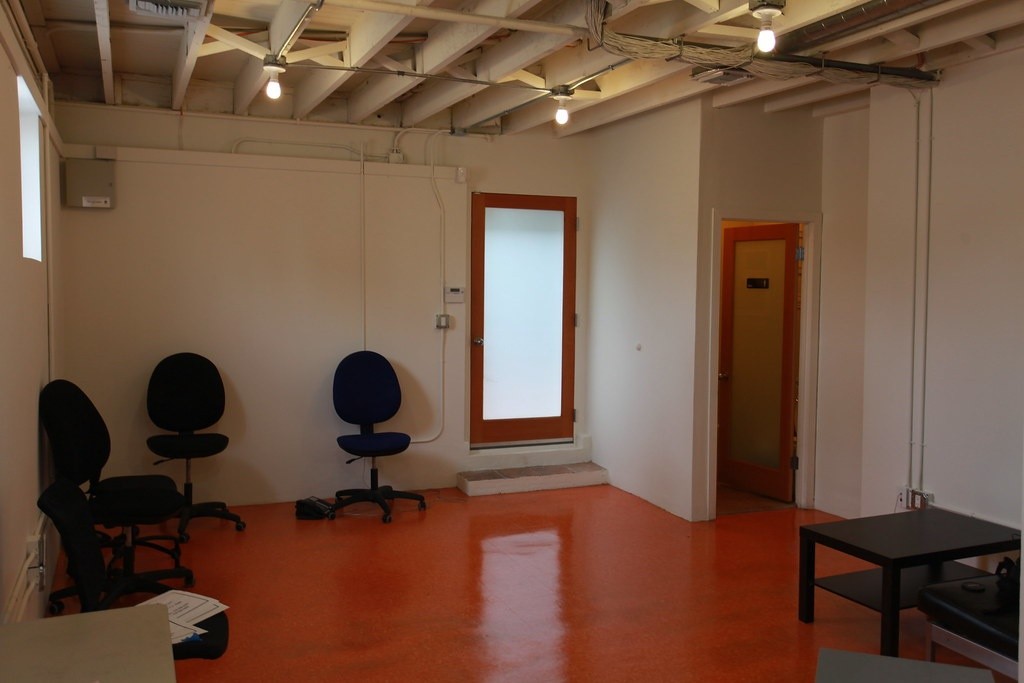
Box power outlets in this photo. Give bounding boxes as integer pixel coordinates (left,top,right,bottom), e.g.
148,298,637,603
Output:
435,314,450,328
895,488,909,508
911,489,934,511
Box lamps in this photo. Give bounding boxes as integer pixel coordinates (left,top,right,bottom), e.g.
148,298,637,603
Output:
262,54,287,99
749,0,787,52
550,85,575,125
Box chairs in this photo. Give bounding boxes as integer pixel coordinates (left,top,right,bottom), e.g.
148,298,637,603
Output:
917,573,1020,683
37,477,229,660
40,380,196,613
324,351,426,523
146,352,247,543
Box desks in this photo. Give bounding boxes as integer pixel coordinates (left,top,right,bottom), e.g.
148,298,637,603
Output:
798,507,1021,657
